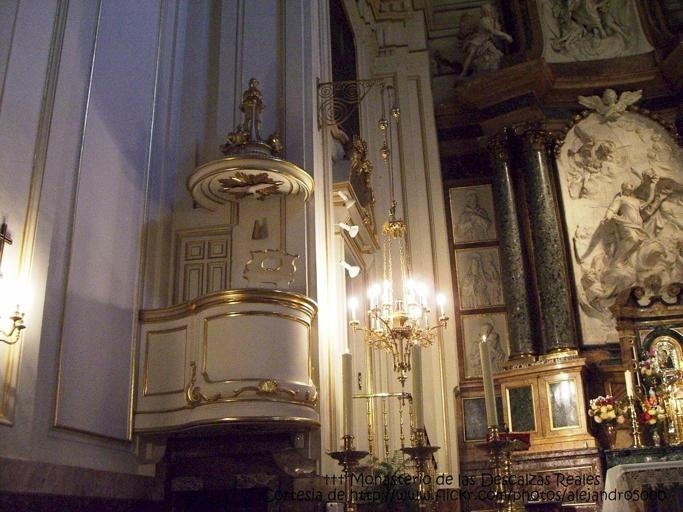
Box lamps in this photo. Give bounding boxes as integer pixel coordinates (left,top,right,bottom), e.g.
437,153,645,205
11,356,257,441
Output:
348,84,452,386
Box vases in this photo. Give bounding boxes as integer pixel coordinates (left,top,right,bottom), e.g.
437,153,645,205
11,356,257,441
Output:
602,423,617,451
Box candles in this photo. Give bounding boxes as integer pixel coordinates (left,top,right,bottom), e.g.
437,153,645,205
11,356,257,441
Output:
411,341,424,432
341,354,353,437
478,335,498,428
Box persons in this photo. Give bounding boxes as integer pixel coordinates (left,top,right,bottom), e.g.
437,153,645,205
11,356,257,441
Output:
461,251,502,305
454,2,517,78
450,188,494,242
236,75,267,137
601,168,668,266
466,323,508,375
550,1,634,53
565,134,613,172
590,89,627,124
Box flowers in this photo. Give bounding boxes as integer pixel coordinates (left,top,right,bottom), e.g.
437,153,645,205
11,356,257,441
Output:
589,393,625,427
